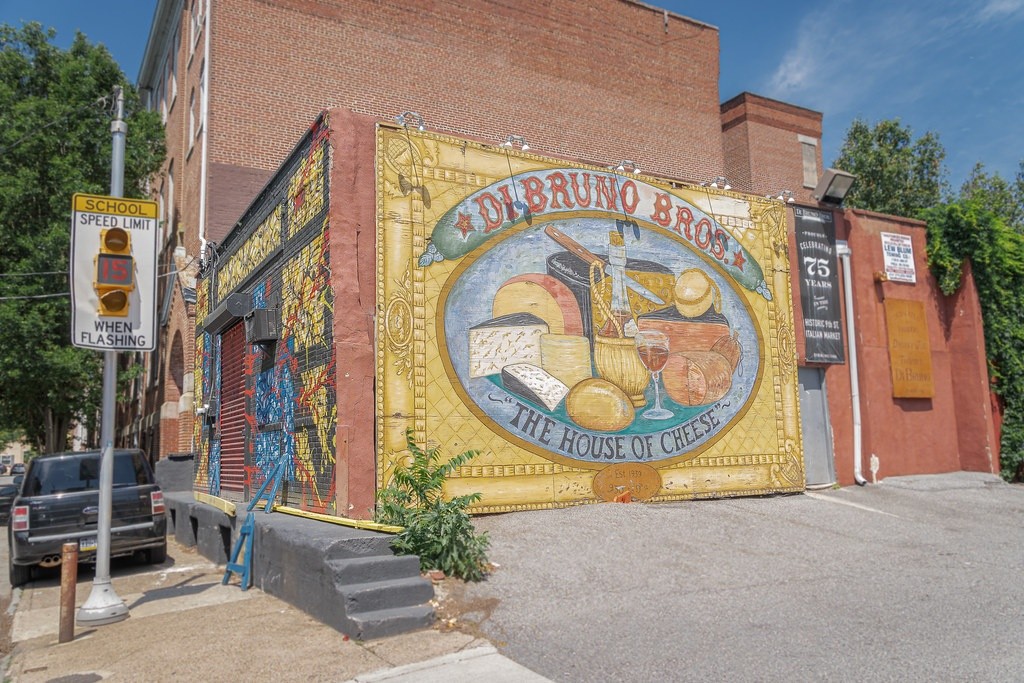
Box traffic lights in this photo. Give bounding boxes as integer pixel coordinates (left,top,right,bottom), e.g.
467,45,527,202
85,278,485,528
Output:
92,227,135,317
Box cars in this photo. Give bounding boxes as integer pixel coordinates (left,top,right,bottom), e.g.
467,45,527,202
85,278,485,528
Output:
0,484,19,521
0,463,7,475
10,463,26,475
8,447,167,585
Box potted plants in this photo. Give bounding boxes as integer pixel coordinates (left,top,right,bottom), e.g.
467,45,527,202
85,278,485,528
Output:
375,426,493,583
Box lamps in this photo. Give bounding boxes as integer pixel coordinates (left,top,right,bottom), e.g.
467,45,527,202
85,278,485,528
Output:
766,190,795,203
607,159,641,175
499,135,530,152
394,110,428,131
700,176,734,191
